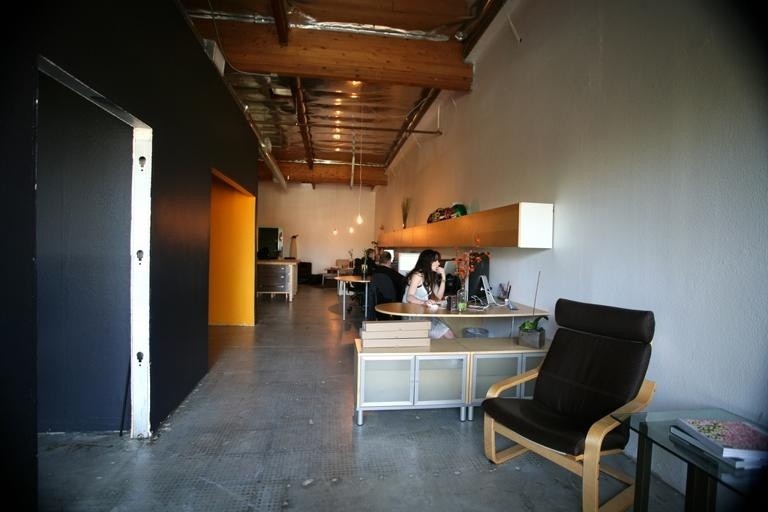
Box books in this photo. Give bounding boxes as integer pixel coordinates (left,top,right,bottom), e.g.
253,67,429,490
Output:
358,320,429,348
670,417,768,470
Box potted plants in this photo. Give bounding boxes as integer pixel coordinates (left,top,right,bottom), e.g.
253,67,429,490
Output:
517,316,550,349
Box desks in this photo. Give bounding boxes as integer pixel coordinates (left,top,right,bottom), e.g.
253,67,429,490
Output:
329,266,373,322
373,300,549,337
255,259,299,303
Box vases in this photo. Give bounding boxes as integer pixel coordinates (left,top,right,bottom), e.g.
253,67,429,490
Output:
457,285,468,312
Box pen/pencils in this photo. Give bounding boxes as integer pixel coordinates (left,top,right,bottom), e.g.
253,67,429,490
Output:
499,280,511,299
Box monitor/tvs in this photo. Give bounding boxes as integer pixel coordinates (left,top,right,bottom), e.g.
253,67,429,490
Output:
398,253,421,277
444,261,463,276
384,249,394,263
464,252,496,305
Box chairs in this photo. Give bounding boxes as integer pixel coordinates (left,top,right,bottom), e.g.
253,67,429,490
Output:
477,299,655,511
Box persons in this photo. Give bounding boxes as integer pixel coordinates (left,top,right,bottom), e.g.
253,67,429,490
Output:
355,248,405,303
402,249,456,339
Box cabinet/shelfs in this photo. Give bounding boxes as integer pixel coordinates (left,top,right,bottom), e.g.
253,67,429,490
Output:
352,337,552,426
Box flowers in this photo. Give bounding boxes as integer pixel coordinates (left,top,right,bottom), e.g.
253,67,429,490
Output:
450,249,491,302
